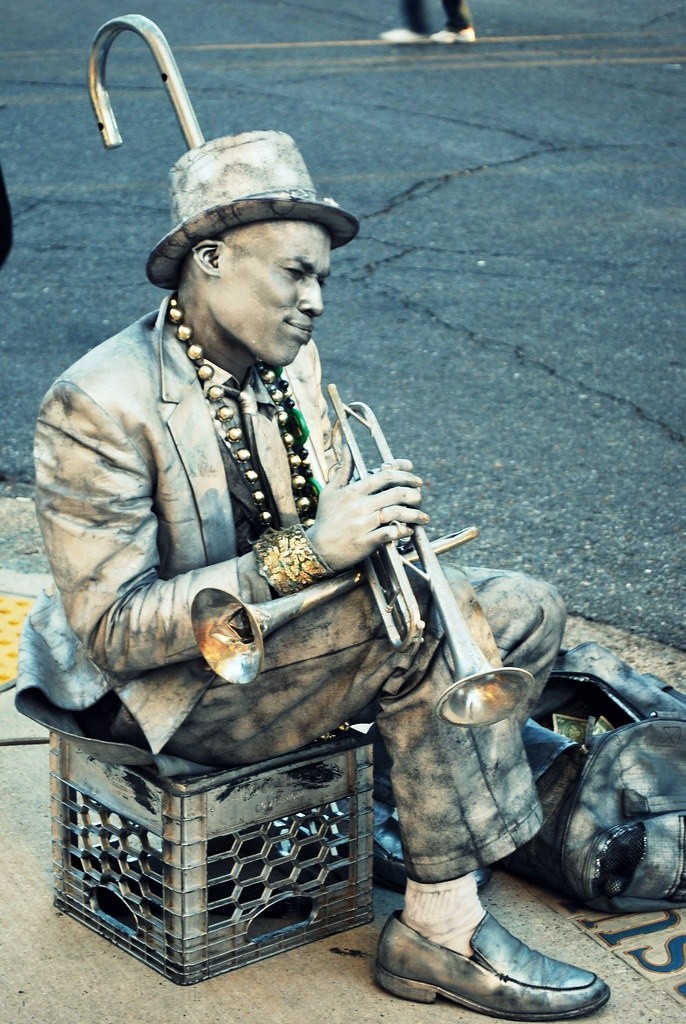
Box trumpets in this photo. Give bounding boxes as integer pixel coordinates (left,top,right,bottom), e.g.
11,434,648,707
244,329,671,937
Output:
321,378,528,730
190,525,480,688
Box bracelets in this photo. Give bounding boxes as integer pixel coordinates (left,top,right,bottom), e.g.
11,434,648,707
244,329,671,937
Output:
254,526,334,597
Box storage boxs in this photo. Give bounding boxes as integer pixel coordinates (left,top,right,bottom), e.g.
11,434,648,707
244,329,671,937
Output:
48,726,374,987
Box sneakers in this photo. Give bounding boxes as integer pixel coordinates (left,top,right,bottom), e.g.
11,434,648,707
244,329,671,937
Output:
429,28,477,44
378,27,432,43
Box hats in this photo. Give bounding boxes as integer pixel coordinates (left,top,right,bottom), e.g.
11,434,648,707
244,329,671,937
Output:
145,129,361,291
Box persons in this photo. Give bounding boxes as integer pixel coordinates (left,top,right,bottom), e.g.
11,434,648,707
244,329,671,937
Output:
381,0,476,43
16,129,612,1022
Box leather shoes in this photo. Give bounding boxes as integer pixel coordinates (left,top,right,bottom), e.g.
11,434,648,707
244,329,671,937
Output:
303,792,492,895
372,908,611,1022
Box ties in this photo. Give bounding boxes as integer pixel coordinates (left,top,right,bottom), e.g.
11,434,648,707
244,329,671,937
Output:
236,384,301,529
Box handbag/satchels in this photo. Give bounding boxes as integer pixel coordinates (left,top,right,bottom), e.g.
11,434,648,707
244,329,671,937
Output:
490,639,686,911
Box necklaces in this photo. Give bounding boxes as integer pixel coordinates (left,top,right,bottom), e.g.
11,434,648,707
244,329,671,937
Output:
168,299,318,528
